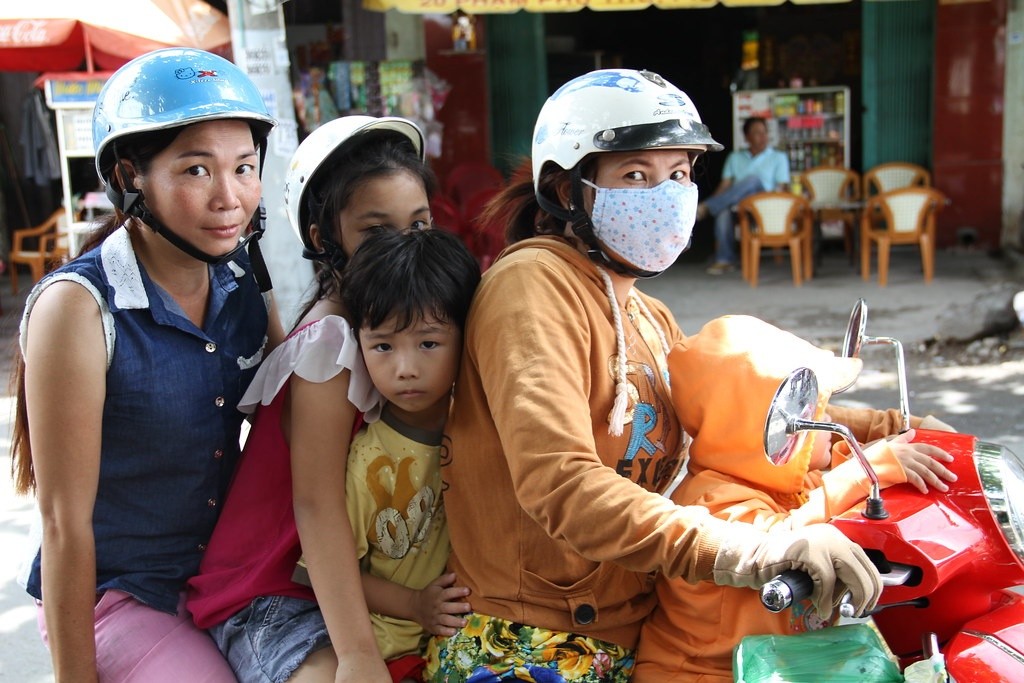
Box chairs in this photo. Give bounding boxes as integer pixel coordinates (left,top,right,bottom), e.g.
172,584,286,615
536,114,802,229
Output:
859,186,947,287
738,192,813,288
799,167,862,276
9,209,81,296
864,162,930,220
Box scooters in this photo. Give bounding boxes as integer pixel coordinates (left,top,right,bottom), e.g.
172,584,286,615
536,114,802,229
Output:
760,299,1024,683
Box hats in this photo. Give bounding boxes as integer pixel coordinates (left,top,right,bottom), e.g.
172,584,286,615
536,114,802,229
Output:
832,356,864,397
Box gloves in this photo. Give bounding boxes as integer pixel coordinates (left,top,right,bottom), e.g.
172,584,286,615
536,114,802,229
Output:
714,519,884,621
918,414,957,432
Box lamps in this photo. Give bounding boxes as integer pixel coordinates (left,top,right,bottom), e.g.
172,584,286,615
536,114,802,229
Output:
448,8,478,55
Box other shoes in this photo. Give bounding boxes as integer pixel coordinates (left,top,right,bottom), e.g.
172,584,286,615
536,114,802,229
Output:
695,203,707,220
708,259,735,275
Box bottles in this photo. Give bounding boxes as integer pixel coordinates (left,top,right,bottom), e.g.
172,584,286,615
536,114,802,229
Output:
774,86,844,176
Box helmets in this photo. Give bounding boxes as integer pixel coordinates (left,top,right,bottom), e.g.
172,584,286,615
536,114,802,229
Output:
284,113,424,257
92,48,276,185
532,69,725,222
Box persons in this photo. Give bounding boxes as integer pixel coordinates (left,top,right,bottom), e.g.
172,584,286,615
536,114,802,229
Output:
629,313,958,683
695,116,790,276
187,114,431,683
8,49,288,682
291,229,482,683
420,68,957,683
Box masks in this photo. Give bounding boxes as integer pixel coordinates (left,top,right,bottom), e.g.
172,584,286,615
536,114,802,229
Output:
568,174,698,273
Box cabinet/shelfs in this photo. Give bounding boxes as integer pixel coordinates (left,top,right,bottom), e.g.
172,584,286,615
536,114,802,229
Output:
732,86,851,174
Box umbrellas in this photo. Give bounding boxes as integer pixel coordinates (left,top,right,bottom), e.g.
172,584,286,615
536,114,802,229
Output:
0,0,233,74
